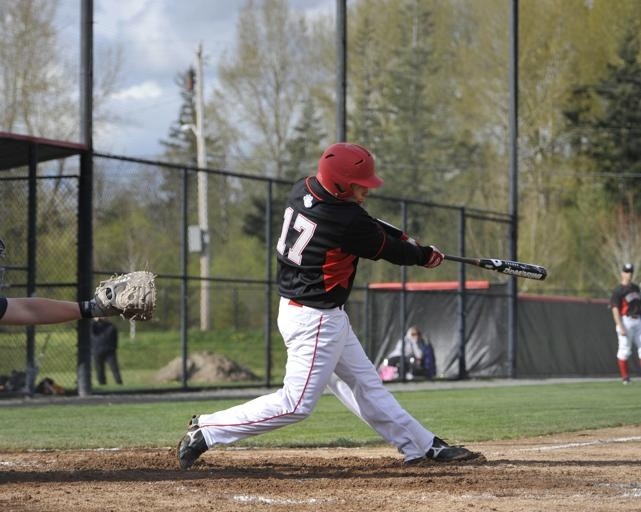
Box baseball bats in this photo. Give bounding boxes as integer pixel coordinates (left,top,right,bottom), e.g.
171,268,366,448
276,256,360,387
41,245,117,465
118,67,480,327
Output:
444,255,547,280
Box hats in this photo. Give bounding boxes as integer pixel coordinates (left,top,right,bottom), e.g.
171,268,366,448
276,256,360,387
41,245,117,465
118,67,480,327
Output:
623,264,633,270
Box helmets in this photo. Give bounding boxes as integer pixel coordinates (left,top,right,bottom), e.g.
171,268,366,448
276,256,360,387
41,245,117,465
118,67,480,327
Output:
316,142,384,200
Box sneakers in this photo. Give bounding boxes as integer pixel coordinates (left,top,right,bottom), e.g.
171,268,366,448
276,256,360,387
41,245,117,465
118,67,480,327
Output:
403,435,471,463
177,413,209,471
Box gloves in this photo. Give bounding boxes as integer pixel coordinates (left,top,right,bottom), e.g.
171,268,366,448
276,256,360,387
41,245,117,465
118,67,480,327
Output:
418,244,445,269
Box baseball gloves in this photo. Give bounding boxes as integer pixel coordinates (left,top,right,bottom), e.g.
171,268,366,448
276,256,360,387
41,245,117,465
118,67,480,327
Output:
90,271,158,320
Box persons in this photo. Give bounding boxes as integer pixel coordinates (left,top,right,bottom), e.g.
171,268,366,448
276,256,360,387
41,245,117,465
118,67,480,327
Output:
411,330,436,381
609,262,641,384
90,316,122,386
0,236,158,325
177,141,476,473
